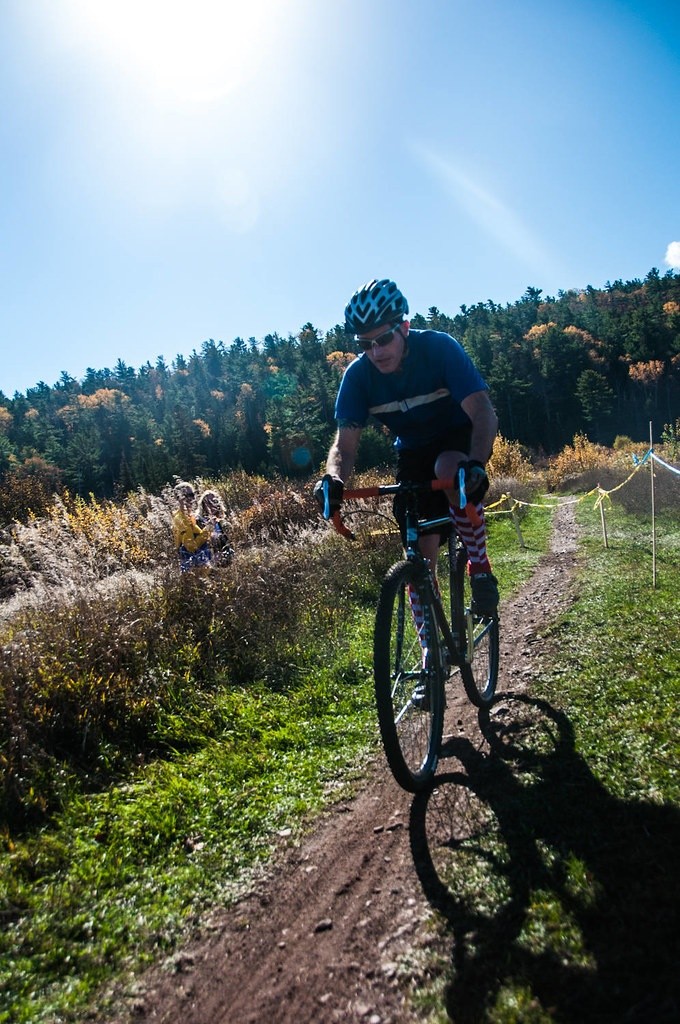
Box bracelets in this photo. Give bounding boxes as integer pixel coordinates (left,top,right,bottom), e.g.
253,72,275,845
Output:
468,460,485,471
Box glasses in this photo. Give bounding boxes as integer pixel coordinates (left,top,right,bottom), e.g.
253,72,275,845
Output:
176,493,194,499
354,322,402,351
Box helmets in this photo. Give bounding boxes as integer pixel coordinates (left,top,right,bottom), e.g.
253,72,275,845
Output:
344,278,409,336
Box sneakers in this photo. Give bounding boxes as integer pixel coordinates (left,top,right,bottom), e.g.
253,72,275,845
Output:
470,572,500,616
411,680,429,711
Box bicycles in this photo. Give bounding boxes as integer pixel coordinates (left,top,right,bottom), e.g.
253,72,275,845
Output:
321,460,499,793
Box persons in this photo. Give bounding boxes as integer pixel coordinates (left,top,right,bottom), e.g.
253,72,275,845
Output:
172,482,234,575
313,279,500,707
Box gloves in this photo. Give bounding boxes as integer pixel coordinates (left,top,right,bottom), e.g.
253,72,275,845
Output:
452,460,490,507
313,475,344,518
